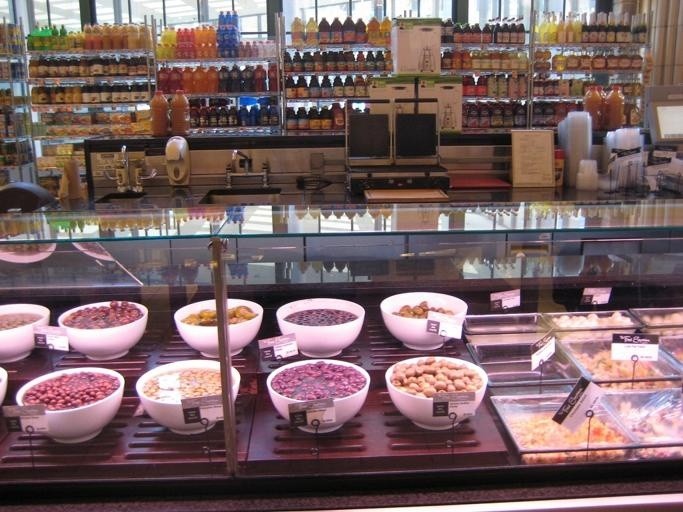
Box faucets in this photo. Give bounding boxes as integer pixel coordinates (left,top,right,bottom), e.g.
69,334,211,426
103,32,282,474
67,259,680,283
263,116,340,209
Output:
223,149,272,189
102,145,159,194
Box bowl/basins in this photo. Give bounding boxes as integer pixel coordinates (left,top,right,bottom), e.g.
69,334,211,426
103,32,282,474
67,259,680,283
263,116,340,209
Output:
266,359,370,434
385,356,488,431
21,367,126,443
276,297,365,359
174,299,263,359
136,360,240,435
58,300,148,361
0,302,50,365
1,231,57,266
72,241,114,260
380,292,468,352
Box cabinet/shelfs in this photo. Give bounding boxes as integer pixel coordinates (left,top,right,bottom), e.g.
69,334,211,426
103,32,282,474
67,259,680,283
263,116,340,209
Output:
530,10,654,137
280,12,530,135
0,198,683,505
0,17,37,190
152,12,283,136
18,16,154,178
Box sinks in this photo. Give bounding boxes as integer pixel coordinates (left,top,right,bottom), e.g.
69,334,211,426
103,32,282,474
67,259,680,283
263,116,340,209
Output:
196,187,284,207
92,191,149,207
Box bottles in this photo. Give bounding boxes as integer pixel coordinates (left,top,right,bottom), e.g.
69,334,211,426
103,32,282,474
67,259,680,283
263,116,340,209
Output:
309,75,321,98
84,22,153,52
463,71,528,98
355,18,368,44
239,41,277,57
344,74,357,97
286,74,296,99
284,52,292,72
533,73,583,96
190,107,199,127
442,46,528,69
169,66,183,91
489,16,525,44
440,18,453,43
228,106,238,126
533,8,632,44
29,52,155,78
229,64,242,92
385,50,393,71
296,107,308,130
188,98,206,108
32,21,68,51
216,11,240,58
357,51,365,71
181,66,194,93
68,31,84,52
218,106,228,127
583,73,642,129
631,13,647,44
286,106,298,130
320,106,332,129
367,17,381,43
380,16,391,43
208,106,218,127
174,28,196,58
240,105,249,127
268,63,277,91
240,97,260,106
158,67,170,92
317,17,330,44
462,98,527,128
532,99,583,128
170,90,190,135
250,106,257,125
305,17,318,45
193,66,206,92
200,106,209,127
302,51,356,71
321,75,331,98
376,51,385,70
355,74,366,98
207,65,218,92
308,106,321,130
0,88,32,166
552,46,643,71
241,64,255,92
32,78,155,104
259,96,278,105
253,64,267,92
330,17,343,44
330,103,345,130
259,105,270,126
149,92,171,138
453,23,491,43
269,105,279,126
534,47,551,71
343,17,358,43
365,74,374,97
292,17,304,46
209,97,234,107
365,51,376,70
292,52,303,72
343,101,371,129
295,76,309,98
155,27,176,59
333,74,344,98
0,202,641,285
217,66,230,91
